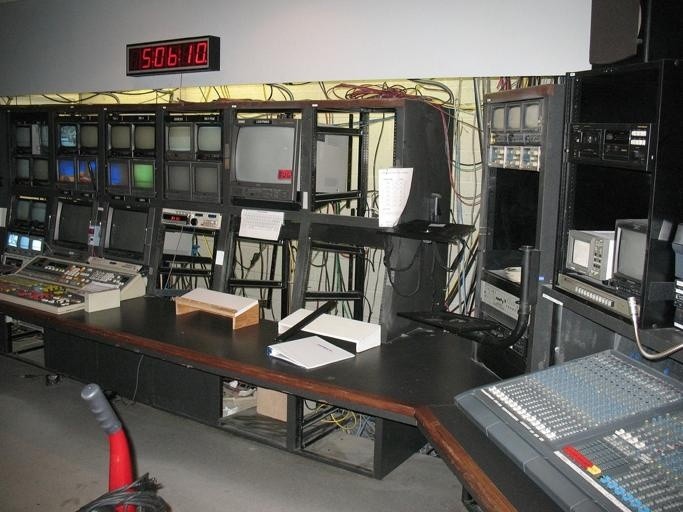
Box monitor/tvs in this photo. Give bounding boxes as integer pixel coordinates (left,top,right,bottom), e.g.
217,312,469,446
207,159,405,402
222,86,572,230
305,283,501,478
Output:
16,200,47,223
13,126,222,194
110,209,148,253
59,203,92,242
229,119,350,204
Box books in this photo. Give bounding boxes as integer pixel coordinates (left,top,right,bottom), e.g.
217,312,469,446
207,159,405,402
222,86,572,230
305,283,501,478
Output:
277,308,382,354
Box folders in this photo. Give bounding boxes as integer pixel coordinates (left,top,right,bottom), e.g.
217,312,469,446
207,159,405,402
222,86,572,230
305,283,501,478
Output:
266,336,355,369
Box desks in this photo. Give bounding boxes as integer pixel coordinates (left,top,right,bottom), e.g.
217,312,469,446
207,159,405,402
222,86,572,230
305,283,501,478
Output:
0,293,558,512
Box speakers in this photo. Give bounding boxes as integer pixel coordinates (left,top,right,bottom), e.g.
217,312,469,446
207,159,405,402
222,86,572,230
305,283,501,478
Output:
589,0,683,69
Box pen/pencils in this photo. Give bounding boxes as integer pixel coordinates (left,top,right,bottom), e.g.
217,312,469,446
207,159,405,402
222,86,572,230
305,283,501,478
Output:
274,299,337,343
